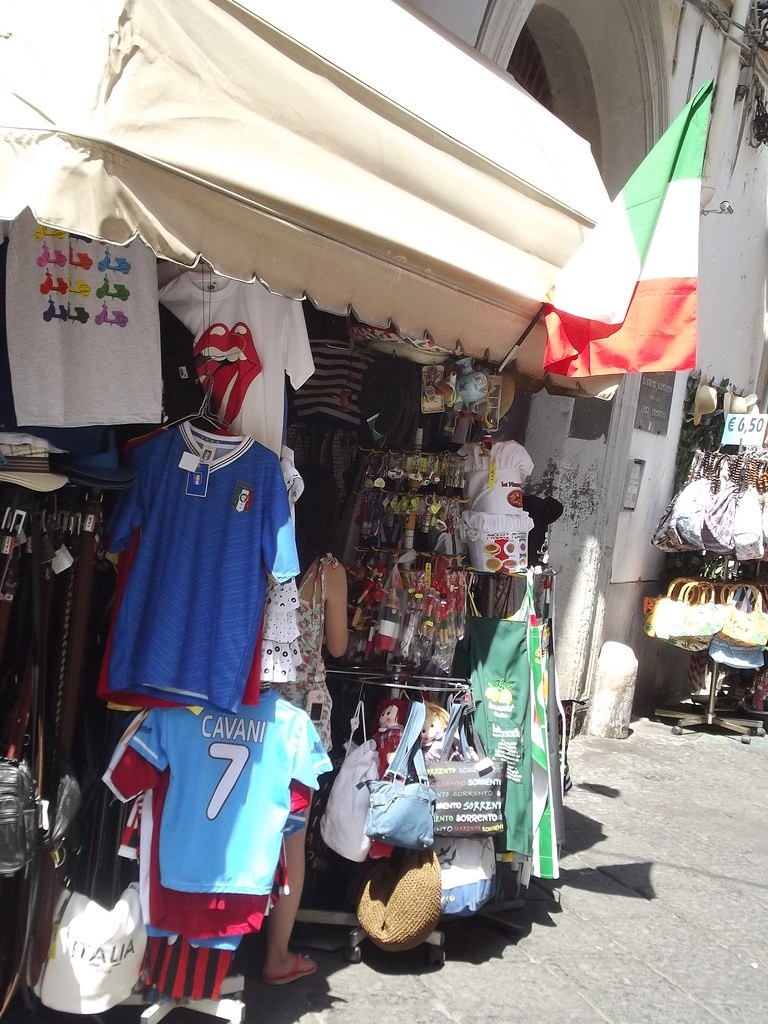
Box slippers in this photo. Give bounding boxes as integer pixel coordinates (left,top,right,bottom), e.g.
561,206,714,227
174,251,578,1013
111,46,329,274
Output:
262,953,318,985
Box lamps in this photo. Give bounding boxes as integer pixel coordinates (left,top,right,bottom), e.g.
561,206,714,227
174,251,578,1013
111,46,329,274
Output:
701,201,734,216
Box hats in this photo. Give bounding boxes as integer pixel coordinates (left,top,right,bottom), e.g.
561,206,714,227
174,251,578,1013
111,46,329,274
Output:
744,393,760,414
0,425,137,493
694,373,717,425
724,383,747,423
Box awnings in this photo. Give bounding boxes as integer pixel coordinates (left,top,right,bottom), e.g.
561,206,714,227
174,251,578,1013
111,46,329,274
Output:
0,0,626,402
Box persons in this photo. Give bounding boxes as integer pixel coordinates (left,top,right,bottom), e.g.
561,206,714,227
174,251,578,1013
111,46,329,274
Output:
259,467,349,984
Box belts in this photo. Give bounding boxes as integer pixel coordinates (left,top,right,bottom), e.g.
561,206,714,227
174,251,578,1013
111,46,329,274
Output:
0,496,106,1021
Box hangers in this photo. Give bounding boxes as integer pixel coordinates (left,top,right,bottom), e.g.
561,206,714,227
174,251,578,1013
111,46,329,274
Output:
161,376,246,440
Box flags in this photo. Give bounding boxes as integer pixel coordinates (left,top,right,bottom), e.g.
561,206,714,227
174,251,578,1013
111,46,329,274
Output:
544,78,713,378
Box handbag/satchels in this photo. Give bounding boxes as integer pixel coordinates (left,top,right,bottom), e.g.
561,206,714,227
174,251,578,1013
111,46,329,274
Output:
356,701,436,851
424,703,507,834
32,882,147,1015
432,835,498,918
651,478,768,561
0,755,40,879
357,850,442,951
319,701,380,862
44,759,82,847
643,577,768,670
